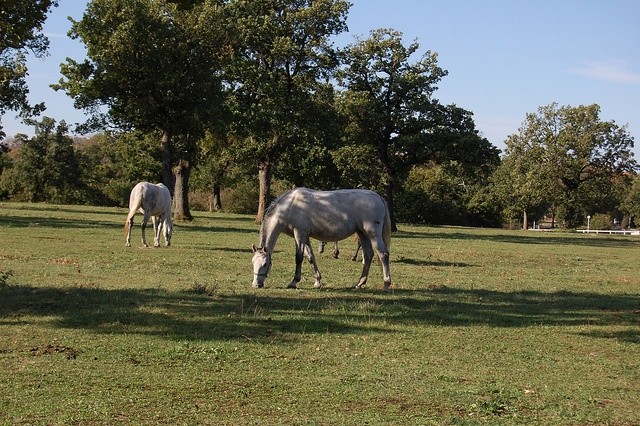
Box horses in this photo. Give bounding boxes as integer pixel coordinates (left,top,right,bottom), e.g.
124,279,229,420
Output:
125,181,174,247
318,232,361,261
252,187,391,292
